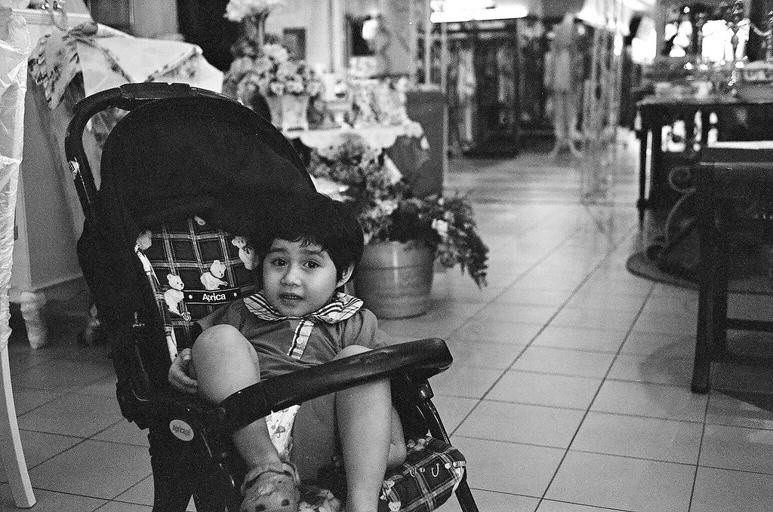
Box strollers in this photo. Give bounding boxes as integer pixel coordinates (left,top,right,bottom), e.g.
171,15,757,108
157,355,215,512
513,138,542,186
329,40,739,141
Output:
65,82,478,512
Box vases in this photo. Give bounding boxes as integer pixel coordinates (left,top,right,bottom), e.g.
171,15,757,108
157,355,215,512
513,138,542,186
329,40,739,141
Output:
265,95,312,134
354,236,438,320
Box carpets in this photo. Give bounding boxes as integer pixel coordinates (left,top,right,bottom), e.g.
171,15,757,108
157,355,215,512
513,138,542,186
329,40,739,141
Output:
624,234,772,296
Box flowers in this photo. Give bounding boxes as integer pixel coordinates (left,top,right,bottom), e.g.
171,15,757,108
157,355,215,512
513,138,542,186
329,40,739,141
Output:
220,0,324,106
305,132,492,291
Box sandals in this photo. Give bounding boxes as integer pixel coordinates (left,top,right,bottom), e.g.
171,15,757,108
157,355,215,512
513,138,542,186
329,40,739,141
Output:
240,463,302,511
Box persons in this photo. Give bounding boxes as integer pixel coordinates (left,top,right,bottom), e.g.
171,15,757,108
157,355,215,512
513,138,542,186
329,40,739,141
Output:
165,191,409,512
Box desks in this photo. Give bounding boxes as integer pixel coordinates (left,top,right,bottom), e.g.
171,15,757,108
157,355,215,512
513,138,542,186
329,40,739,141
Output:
688,140,771,395
